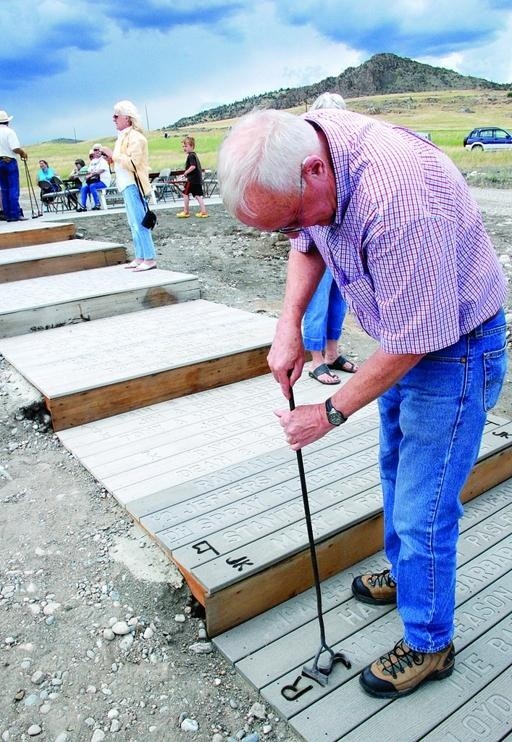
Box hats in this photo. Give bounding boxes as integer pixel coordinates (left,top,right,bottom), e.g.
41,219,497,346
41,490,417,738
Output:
0,110,14,123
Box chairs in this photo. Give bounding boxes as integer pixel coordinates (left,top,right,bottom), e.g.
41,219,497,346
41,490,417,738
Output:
35,168,220,216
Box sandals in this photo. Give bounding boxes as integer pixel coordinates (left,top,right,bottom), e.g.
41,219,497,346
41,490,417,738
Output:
124,257,159,272
306,354,364,385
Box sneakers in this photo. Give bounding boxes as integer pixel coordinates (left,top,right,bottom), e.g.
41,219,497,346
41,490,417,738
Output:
176,211,190,219
349,569,406,607
76,206,87,212
7,215,28,222
91,205,102,210
355,631,457,701
194,211,209,218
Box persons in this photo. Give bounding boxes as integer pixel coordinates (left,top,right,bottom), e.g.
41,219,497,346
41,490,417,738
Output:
75,143,111,213
214,105,509,701
0,110,30,224
36,159,62,206
97,100,158,272
65,158,87,210
299,92,361,386
175,136,207,218
88,148,94,161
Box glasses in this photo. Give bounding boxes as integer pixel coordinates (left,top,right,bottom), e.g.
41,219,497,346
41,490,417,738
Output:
113,114,132,118
272,153,309,234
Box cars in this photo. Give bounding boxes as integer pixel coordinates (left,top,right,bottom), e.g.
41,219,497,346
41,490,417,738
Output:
463,125,512,152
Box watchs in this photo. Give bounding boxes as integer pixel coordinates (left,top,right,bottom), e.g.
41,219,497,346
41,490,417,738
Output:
325,397,348,428
